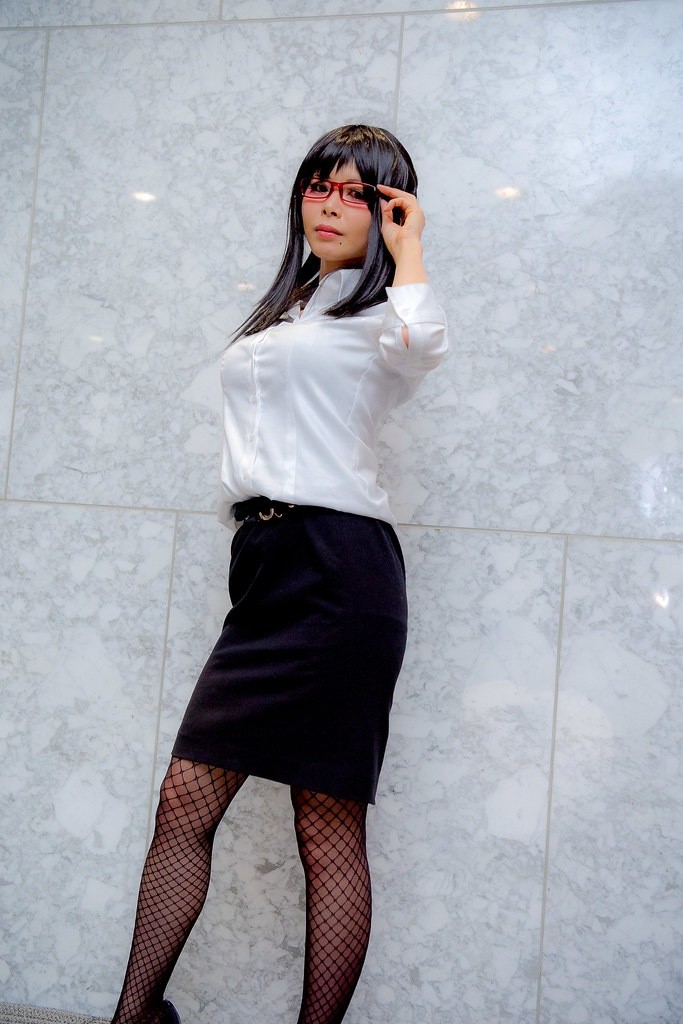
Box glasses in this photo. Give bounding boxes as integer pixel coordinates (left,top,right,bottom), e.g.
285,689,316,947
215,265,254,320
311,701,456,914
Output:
299,176,381,205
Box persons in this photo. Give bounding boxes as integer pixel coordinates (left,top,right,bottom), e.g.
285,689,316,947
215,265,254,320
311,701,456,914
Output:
108,124,447,1024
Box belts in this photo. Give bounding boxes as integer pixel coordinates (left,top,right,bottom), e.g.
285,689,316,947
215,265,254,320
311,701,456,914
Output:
234,495,298,522
